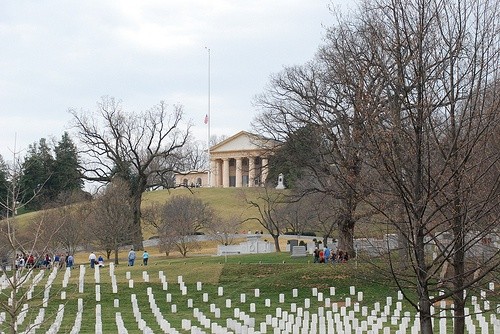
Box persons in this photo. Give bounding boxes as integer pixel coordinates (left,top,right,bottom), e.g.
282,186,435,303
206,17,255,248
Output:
89,252,96,268
15,253,75,270
98,256,104,267
143,251,148,265
127,248,136,266
314,246,350,264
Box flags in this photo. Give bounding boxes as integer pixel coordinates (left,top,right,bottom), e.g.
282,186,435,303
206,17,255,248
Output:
204,114,209,124
